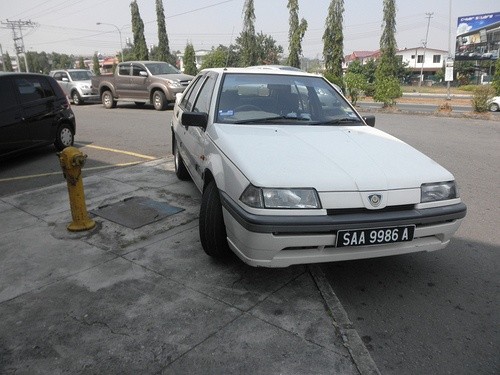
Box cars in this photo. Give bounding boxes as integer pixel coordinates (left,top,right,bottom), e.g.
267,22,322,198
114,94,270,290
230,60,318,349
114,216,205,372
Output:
172,65,466,269
487,96,500,112
0,73,75,162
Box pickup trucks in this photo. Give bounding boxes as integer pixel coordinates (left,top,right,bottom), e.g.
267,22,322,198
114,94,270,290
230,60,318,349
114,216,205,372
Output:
92,61,195,110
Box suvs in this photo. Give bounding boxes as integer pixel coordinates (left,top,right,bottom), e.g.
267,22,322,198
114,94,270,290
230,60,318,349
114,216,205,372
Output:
49,69,96,105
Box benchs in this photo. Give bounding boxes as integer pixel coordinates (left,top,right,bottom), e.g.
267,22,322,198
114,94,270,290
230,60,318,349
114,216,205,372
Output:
236,95,269,107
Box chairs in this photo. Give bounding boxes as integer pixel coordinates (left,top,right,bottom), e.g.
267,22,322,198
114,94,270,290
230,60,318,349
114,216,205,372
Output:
268,92,300,117
216,89,241,113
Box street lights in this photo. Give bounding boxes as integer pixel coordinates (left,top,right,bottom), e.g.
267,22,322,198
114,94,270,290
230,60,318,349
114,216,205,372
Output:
97,22,124,62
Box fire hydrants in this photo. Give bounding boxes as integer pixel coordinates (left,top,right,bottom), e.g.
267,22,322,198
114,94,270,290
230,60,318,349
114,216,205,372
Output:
56,147,94,231
